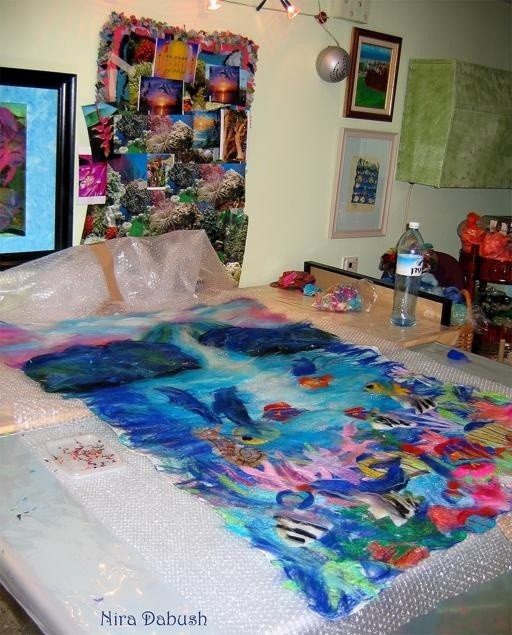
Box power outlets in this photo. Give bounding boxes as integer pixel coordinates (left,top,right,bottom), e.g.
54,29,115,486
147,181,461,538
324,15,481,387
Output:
342,256,358,274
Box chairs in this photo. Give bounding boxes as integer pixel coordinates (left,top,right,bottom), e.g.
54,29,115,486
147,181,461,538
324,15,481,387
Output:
457,215,512,303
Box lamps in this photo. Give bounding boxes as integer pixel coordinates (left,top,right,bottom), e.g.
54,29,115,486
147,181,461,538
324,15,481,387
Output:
256,0,302,19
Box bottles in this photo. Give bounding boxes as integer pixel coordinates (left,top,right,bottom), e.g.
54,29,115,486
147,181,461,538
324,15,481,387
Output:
389,219,426,331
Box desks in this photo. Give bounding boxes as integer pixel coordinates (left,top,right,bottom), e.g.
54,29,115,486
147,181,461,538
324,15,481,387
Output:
0,293,511,635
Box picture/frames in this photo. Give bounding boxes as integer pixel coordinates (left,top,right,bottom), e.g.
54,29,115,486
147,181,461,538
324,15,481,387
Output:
328,128,400,240
0,66,78,270
342,26,403,123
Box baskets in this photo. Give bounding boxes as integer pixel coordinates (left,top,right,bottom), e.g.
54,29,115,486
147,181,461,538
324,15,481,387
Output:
454,289,474,353
